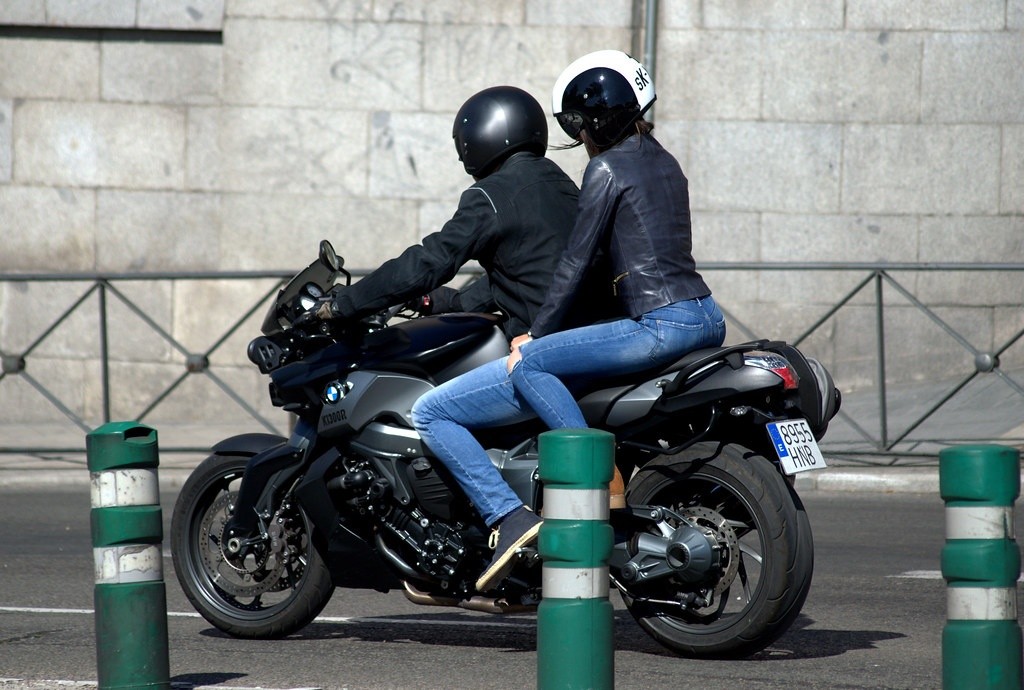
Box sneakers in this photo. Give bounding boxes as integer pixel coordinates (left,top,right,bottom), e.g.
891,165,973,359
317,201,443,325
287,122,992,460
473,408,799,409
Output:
475,504,544,591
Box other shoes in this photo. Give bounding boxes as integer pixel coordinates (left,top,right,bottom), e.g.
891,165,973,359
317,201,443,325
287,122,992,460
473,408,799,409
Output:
608,465,626,508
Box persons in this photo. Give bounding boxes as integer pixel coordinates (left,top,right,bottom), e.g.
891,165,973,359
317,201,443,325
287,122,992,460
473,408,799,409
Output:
507,49,726,509
312,87,607,591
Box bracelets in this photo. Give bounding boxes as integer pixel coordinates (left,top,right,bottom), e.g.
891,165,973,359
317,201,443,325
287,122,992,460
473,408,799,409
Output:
527,331,537,340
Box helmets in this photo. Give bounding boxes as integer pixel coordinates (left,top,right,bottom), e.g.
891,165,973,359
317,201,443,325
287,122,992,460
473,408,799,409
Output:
551,49,656,151
453,86,548,184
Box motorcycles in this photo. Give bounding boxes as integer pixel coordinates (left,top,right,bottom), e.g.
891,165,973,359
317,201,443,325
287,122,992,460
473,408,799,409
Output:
170,240,843,660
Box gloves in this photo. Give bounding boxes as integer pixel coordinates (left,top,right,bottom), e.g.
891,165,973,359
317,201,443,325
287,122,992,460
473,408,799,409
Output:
293,302,337,350
